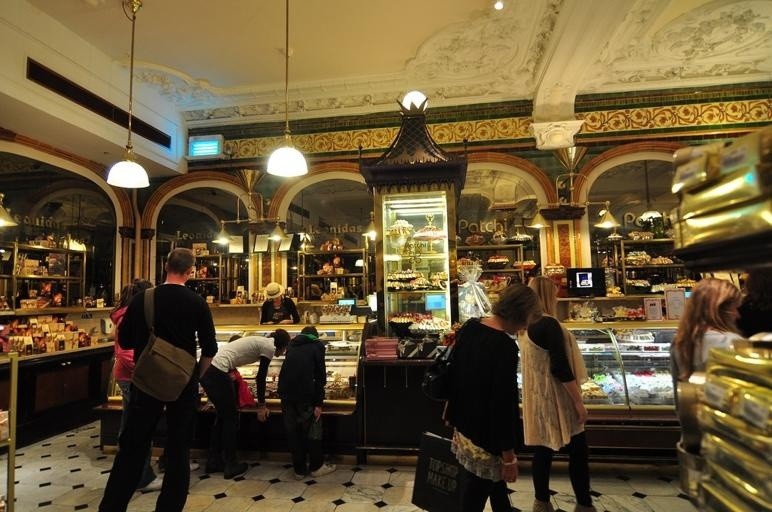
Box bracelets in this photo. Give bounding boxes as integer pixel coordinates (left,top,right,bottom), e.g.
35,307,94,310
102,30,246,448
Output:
499,457,518,465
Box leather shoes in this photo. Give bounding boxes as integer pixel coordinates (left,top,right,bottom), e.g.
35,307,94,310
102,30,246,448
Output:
206,463,224,473
189,462,199,470
224,462,248,478
140,475,165,493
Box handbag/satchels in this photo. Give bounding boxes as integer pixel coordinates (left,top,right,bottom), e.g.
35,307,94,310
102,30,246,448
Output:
410,420,491,509
131,286,197,403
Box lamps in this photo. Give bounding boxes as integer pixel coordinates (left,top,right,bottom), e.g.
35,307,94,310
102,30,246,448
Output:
212,169,291,243
526,146,621,228
105,1,150,188
266,0,308,179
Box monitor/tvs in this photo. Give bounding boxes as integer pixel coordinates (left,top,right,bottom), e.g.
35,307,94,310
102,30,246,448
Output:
566,267,607,300
337,298,356,305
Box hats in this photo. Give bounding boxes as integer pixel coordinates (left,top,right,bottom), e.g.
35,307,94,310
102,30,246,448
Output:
264,282,284,298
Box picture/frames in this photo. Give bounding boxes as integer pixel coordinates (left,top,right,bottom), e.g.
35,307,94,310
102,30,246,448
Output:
254,234,270,253
229,234,243,253
192,243,207,258
278,234,294,251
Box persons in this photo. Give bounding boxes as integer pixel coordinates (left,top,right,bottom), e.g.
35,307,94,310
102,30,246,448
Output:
444,284,543,511
200,329,291,480
260,282,300,325
97,249,219,512
519,276,596,511
278,326,337,480
671,278,743,382
110,279,199,495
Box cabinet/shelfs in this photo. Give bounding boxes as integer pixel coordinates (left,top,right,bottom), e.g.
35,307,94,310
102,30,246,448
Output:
0,342,114,456
298,248,368,301
355,359,453,466
0,233,86,315
614,238,696,295
373,181,458,337
508,323,681,465
457,245,524,284
555,295,687,324
94,325,365,455
0,353,18,512
161,253,239,303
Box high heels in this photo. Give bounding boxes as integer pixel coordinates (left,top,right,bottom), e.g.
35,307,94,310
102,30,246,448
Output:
531,499,556,512
573,503,599,512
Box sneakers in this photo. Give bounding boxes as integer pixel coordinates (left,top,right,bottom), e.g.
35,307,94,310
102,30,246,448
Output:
294,471,304,480
310,462,336,478
